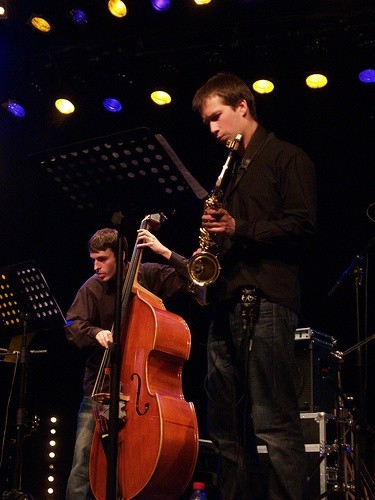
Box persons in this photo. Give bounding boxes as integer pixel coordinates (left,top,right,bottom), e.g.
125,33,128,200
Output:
192,74,317,500
62,229,197,500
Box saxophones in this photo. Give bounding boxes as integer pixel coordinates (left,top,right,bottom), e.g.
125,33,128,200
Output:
183,132,245,306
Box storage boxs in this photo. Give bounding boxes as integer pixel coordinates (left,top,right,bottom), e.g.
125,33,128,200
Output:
250,327,356,500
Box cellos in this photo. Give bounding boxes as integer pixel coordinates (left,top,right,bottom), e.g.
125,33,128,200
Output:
86,213,200,500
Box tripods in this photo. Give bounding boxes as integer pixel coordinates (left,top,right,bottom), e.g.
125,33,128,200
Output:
310,333,375,500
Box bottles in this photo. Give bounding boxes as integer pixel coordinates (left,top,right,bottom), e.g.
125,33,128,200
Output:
189,482,207,500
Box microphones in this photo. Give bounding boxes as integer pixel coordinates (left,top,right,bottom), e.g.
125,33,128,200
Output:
328,256,360,297
